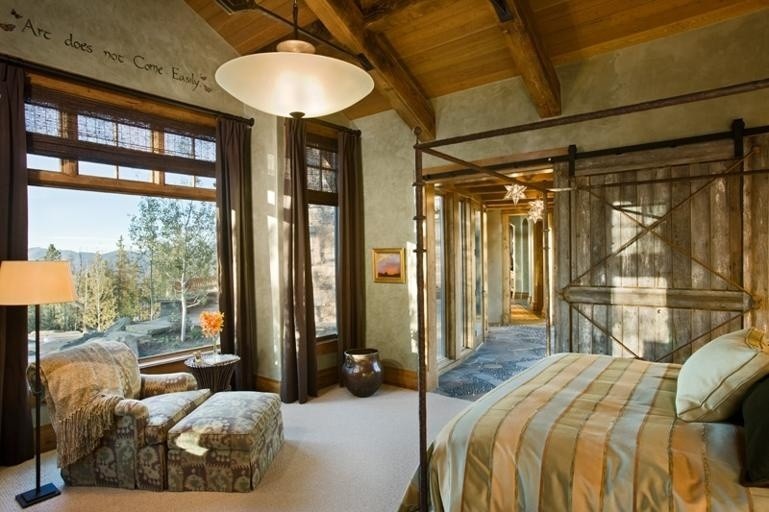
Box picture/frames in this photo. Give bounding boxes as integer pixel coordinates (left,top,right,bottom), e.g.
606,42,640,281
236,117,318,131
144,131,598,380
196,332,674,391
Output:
372,247,406,284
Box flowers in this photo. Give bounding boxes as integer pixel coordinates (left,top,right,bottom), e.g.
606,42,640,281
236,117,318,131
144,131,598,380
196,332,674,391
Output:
199,310,225,338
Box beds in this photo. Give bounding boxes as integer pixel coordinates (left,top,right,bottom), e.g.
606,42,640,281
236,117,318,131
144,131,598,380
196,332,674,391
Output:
425,352,769,512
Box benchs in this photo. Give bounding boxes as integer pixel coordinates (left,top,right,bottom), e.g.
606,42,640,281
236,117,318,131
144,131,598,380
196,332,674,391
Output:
167,390,285,494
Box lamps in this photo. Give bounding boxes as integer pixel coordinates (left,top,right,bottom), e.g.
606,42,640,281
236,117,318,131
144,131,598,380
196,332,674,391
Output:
215,0,375,121
0,259,79,509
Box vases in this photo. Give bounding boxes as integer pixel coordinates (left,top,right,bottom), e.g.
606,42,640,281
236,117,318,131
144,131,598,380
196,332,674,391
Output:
213,336,218,358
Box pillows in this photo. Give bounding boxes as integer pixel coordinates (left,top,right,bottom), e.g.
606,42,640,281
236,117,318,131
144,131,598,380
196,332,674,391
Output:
674,327,769,488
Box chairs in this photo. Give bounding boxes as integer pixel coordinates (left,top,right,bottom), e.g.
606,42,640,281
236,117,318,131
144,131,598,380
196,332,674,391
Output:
25,337,212,493
